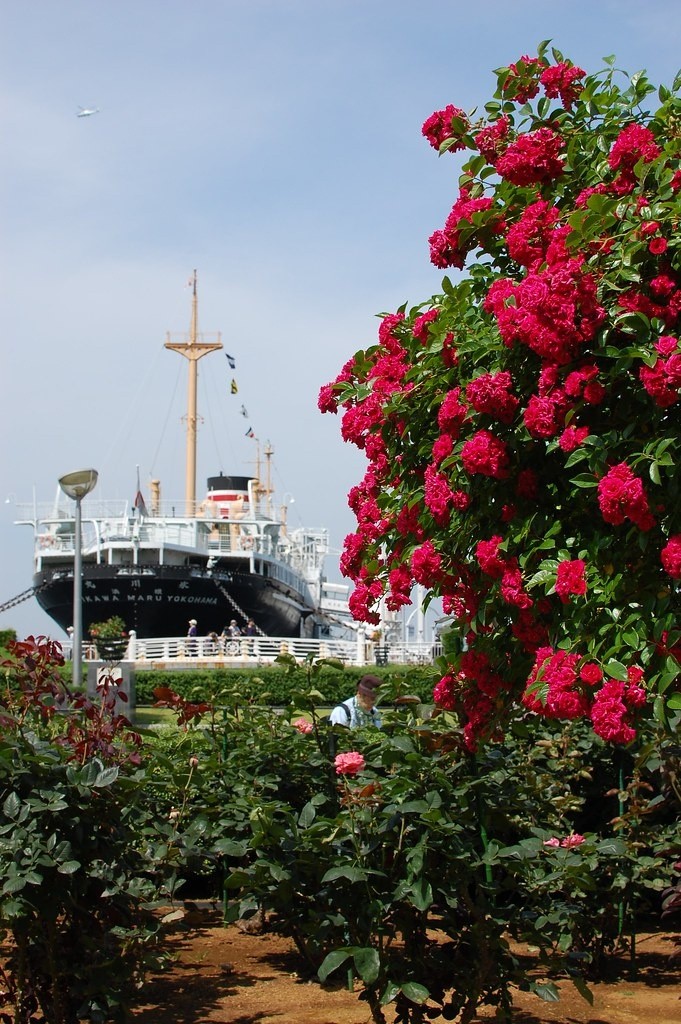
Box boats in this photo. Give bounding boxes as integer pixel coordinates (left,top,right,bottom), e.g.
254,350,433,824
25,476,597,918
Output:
7,262,334,642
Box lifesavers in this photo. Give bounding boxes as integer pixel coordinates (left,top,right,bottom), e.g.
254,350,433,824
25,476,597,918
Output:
40,535,53,548
240,536,253,548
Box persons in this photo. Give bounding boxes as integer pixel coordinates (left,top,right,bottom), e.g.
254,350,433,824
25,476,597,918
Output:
185,619,197,656
205,619,256,656
327,675,384,731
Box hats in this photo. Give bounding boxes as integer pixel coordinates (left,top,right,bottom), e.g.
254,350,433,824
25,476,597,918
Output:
188,619,197,624
358,675,384,697
209,556,214,558
230,620,237,624
67,627,73,631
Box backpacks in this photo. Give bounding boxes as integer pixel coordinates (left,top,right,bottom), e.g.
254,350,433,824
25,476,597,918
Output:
317,703,374,762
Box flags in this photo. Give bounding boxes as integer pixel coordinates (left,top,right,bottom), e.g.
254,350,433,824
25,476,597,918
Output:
230,379,238,394
240,405,249,418
226,354,236,369
245,427,256,439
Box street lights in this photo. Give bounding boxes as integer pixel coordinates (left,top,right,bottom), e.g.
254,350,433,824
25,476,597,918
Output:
58,468,101,690
